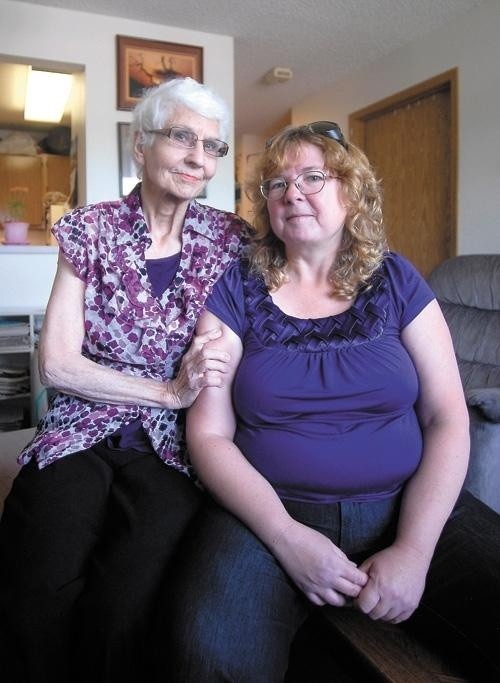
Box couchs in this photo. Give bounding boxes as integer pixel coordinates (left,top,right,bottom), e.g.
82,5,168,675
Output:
427,255,500,391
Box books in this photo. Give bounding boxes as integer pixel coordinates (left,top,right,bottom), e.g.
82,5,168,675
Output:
1,319,31,433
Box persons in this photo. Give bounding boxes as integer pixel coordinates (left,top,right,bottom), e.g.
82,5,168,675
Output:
1,74,258,679
163,117,499,681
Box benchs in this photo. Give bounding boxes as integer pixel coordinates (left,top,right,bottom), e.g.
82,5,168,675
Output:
0,426,465,683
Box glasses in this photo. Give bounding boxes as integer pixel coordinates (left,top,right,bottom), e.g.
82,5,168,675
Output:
259,171,341,200
148,126,229,157
266,121,348,150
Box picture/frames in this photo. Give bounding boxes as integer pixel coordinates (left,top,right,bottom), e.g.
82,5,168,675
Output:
116,33,204,111
117,121,207,198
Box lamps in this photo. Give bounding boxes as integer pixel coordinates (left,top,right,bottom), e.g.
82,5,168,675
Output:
23,64,73,124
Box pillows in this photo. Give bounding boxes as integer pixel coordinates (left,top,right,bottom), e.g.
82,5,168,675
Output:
463,387,500,514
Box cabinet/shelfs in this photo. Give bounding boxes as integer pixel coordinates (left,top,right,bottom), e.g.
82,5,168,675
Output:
0,151,70,230
0,305,59,427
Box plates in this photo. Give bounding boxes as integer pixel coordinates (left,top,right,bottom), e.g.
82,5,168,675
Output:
1,239,32,246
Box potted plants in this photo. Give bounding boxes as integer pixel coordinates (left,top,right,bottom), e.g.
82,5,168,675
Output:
1,188,30,245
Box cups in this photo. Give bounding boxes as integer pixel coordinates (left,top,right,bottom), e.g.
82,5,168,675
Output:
3,218,30,242
